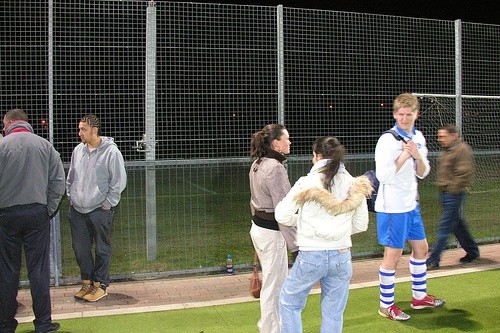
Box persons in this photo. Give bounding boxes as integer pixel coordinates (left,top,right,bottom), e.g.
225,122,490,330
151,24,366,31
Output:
1,108,66,332
427,124,481,269
248,124,298,333
66,113,127,302
374,91,445,320
274,134,370,333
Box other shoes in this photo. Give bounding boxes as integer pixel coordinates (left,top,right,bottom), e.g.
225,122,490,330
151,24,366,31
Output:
460,255,480,262
426,260,439,270
46,322,60,333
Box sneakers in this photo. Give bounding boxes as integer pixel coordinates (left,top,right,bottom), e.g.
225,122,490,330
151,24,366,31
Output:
377,304,410,321
410,294,445,310
74,280,108,302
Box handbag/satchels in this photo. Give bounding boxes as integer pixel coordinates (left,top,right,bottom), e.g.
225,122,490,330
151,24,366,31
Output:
249,250,264,298
359,168,380,212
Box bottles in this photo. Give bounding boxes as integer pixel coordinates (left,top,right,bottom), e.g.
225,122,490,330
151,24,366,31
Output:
226,255,233,276
